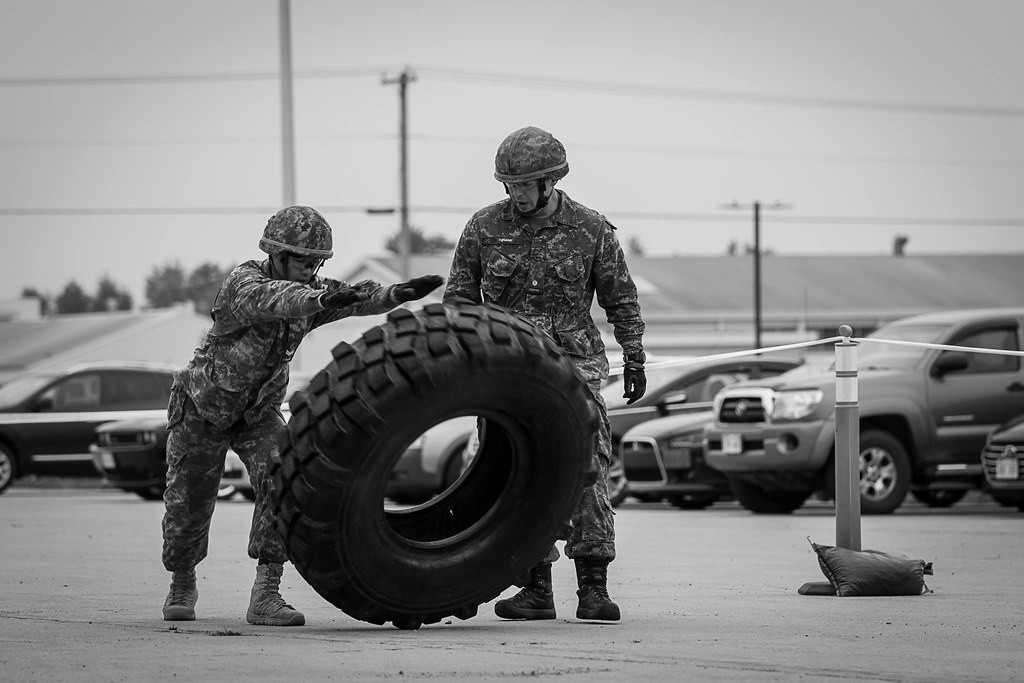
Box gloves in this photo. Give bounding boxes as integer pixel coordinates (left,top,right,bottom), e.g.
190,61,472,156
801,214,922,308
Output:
320,285,370,311
394,274,444,303
622,367,646,405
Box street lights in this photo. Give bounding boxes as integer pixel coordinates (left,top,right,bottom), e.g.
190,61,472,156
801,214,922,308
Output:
380,69,418,280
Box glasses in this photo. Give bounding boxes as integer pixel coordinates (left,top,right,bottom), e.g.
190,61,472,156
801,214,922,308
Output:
505,180,537,189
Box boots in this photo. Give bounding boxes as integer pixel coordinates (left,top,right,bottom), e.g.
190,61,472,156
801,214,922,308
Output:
574,556,620,620
495,563,556,619
162,570,198,622
247,561,305,626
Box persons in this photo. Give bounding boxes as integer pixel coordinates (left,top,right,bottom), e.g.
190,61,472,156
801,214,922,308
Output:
443,125,648,619
158,205,443,626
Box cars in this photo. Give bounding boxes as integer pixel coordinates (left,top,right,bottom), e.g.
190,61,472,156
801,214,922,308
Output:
601,310,1024,517
0,358,297,503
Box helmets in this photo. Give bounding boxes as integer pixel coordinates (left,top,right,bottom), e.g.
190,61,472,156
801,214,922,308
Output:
493,126,569,183
259,206,334,260
